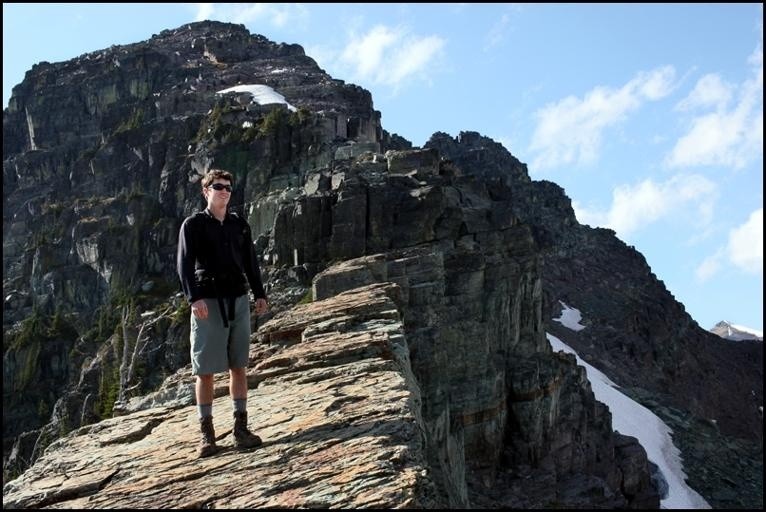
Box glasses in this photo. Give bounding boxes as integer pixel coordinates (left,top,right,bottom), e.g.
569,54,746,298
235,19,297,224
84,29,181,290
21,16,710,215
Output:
208,183,233,192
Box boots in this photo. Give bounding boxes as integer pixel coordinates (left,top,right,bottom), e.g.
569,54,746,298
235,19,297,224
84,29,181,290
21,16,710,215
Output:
197,415,216,457
233,410,262,448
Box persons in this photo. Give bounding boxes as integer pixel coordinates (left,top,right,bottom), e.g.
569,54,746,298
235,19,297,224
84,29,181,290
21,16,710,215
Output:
176,168,268,459
